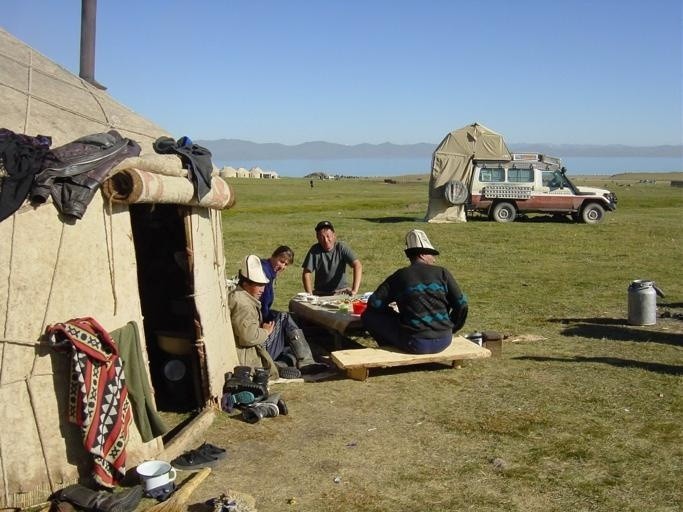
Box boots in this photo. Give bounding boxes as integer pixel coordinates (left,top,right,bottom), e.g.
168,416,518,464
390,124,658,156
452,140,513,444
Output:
275,329,329,378
222,367,288,424
57,484,142,512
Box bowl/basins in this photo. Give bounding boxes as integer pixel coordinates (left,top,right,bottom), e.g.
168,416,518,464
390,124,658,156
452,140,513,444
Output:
297,292,310,301
307,295,321,306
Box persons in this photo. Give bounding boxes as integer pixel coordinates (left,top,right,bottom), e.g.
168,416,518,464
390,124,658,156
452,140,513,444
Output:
227,245,327,377
303,222,360,295
360,228,467,354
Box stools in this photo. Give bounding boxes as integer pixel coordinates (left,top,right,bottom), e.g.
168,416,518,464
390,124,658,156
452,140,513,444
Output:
330,334,493,380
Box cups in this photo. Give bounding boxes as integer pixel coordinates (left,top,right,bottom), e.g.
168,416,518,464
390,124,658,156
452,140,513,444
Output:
135,459,178,495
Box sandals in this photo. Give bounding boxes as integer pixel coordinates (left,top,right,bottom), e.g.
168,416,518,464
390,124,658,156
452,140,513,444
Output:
168,444,226,470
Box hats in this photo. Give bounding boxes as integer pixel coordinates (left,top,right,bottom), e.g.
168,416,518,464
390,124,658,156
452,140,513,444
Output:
237,253,270,284
403,230,439,255
316,220,333,231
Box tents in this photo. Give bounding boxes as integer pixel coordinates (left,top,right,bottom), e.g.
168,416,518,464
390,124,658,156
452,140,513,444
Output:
426,122,513,223
0,27,237,512
221,166,278,179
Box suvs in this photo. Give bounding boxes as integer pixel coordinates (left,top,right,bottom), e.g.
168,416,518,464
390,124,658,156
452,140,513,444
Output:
445,152,618,225
